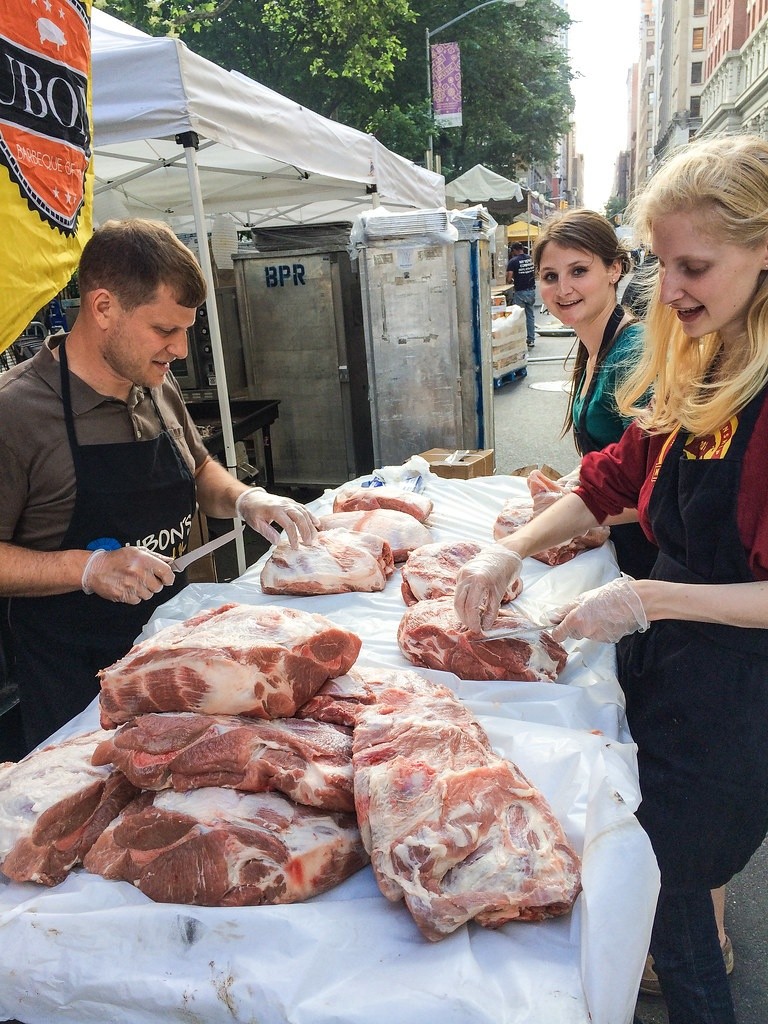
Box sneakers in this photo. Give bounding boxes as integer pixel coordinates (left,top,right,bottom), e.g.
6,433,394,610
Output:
526,340,535,347
640,933,735,998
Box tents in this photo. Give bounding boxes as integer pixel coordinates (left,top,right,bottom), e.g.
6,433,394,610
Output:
446,163,539,256
91,7,446,580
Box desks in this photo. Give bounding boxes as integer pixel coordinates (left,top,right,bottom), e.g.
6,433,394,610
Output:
180,400,281,584
491,284,515,300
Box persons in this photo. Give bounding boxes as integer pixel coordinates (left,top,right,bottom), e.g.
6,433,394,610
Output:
532,208,658,458
453,131,768,1024
620,253,661,318
0,217,320,766
505,242,537,348
630,249,642,268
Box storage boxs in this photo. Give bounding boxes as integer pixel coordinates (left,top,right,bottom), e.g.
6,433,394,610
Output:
511,464,564,481
404,447,494,480
491,308,529,389
